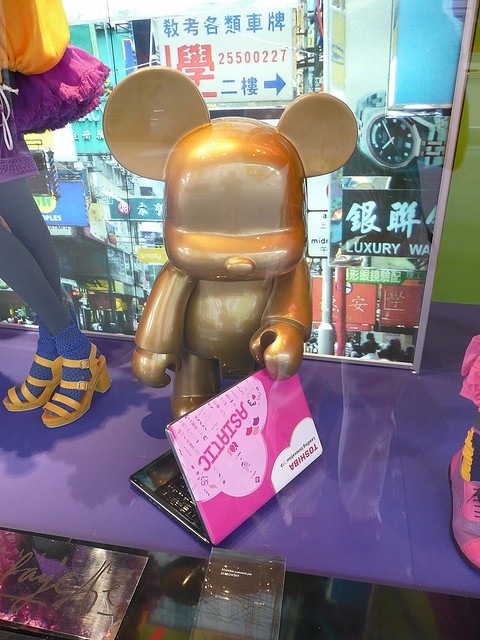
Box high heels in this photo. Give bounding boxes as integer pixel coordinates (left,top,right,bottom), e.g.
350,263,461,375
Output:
41,342,112,429
3,354,62,413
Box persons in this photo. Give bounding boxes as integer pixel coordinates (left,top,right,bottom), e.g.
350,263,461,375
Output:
344,333,414,363
0,0,110,428
447,334,480,573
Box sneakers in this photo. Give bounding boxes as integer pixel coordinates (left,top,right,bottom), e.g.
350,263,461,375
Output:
448,427,480,575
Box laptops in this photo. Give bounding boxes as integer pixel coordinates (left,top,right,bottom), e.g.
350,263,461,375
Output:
129,367,325,546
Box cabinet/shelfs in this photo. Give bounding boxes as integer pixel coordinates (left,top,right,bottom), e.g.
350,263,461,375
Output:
0,303,479,602
